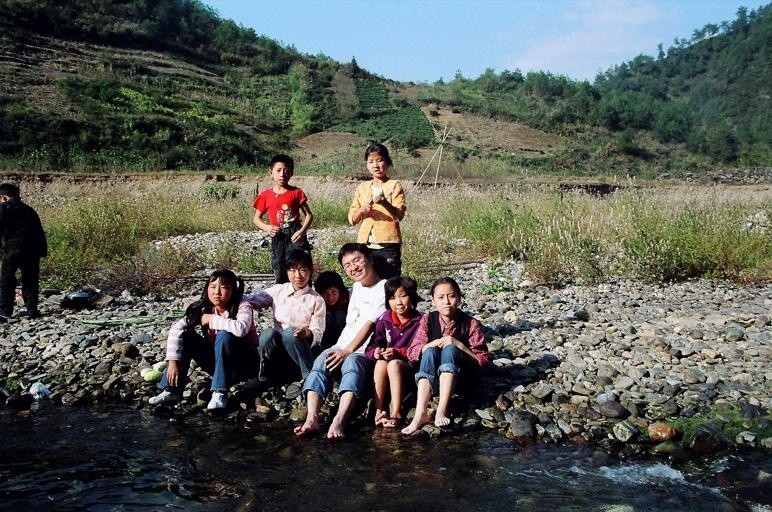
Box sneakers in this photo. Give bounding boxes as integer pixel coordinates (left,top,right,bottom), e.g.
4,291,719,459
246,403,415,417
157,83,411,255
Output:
207,393,226,410
148,390,182,405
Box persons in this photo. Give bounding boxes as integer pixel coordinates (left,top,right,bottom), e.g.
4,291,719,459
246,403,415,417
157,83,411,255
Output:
248,153,316,289
313,269,351,361
346,142,408,281
241,247,329,397
291,242,389,441
0,182,50,325
362,275,423,430
398,276,491,435
145,268,259,412
